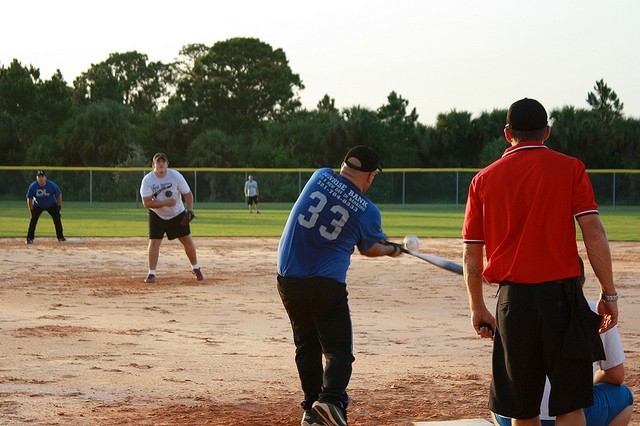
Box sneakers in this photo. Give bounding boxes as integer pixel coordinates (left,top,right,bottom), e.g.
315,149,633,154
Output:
191,267,203,280
300,410,326,426
146,274,155,283
311,396,349,426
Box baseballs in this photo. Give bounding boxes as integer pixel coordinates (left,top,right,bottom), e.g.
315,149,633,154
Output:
404,235,420,250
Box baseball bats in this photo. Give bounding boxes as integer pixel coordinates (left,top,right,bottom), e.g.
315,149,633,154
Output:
400,247,496,287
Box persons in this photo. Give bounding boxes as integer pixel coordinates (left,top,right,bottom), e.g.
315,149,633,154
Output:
139,152,204,283
488,255,633,426
25,170,67,244
274,144,403,426
244,175,260,214
460,96,619,426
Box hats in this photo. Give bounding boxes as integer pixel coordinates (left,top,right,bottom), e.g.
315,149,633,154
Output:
37,170,45,176
153,152,169,163
506,98,548,131
344,145,382,173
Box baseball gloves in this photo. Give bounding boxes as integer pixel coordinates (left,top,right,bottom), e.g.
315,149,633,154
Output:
180,211,194,225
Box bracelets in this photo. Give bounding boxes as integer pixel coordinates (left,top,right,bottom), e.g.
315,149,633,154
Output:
600,292,618,302
387,245,397,256
